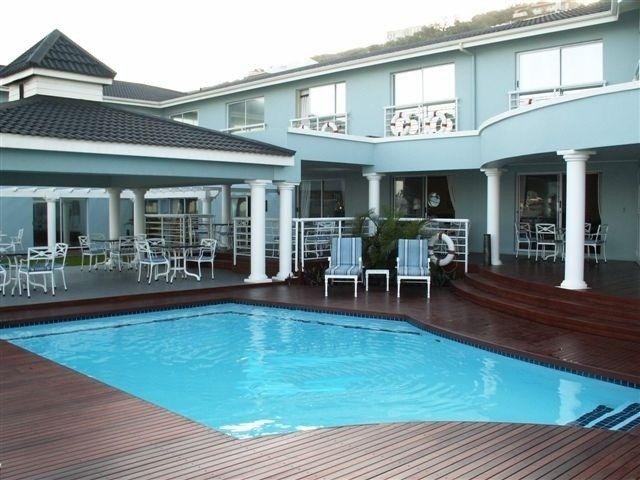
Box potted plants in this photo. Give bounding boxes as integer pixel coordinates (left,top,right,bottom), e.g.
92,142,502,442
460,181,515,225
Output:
354,208,433,279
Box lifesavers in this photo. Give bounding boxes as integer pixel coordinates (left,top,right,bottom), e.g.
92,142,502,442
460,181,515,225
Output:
428,232,455,267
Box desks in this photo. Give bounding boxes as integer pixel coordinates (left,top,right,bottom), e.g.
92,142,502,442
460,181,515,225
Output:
362,263,390,292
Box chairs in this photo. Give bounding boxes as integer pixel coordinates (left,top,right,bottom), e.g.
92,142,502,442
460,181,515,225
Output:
1,227,218,301
322,237,366,298
394,238,432,300
513,219,609,265
268,212,466,260
